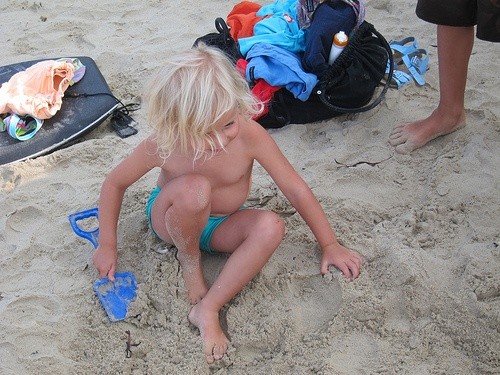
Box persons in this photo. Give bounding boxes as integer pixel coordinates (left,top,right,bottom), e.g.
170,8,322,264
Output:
387,0,500,154
93,40,364,364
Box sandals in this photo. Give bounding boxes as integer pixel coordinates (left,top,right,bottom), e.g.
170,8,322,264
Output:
381,48,430,90
375,35,419,83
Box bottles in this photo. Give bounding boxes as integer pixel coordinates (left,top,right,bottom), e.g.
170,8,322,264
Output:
328,30,348,64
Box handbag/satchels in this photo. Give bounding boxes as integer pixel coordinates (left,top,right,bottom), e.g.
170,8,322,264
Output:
214,7,394,125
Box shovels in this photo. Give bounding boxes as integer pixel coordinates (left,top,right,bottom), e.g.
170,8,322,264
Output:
68,207,139,324
